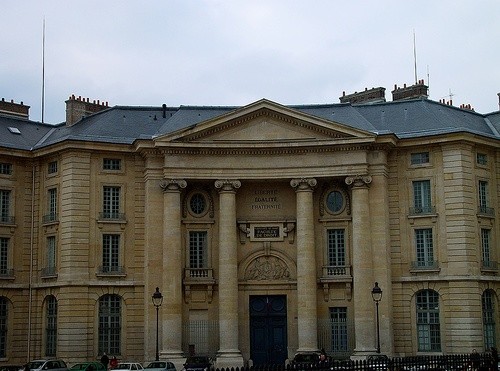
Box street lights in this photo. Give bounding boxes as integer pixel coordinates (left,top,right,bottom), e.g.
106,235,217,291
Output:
151,286,164,360
371,281,383,353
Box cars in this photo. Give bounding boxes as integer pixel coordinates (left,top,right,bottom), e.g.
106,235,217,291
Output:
109,362,145,371
0,365,21,371
329,359,354,371
143,360,177,371
66,362,107,371
402,354,500,371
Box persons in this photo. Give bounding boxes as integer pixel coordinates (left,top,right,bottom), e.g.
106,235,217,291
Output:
100,352,109,371
24,364,30,371
319,349,328,362
470,347,498,371
110,356,118,367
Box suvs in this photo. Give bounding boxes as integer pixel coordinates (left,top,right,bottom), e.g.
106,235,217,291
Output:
289,353,322,371
182,356,211,371
18,358,68,371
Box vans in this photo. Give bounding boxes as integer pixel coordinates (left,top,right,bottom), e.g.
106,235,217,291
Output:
366,354,390,371
